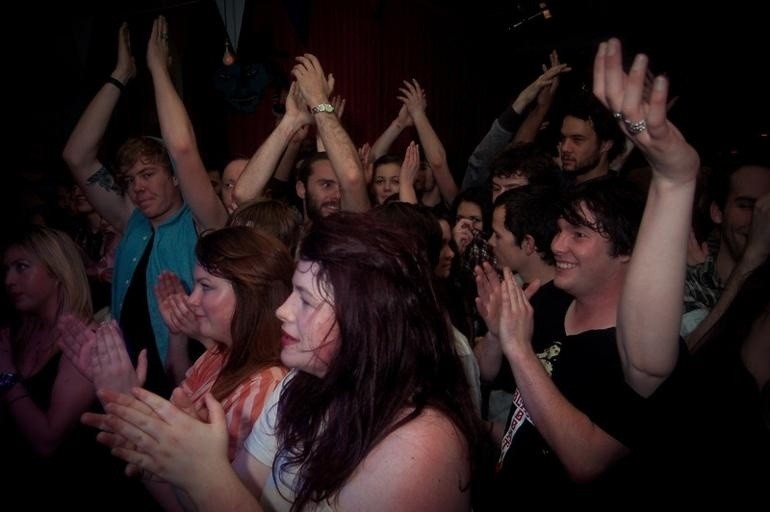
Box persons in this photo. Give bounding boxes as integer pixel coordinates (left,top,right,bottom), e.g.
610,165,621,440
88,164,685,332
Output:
0,13,769,512
